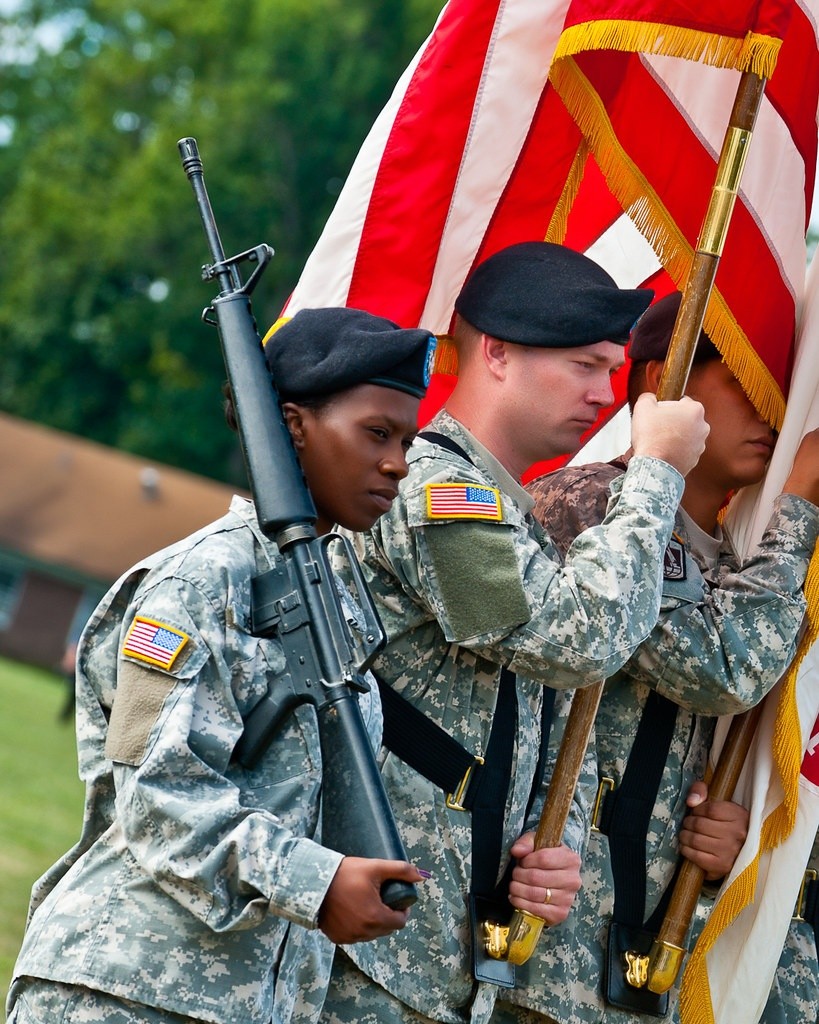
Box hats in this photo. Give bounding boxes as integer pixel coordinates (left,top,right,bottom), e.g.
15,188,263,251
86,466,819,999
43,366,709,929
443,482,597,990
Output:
266,307,440,400
632,291,720,361
454,241,659,348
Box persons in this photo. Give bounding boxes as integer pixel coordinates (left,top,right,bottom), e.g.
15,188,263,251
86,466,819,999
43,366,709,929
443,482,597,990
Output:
526,291,819,1024
5,307,444,1024
322,237,715,1024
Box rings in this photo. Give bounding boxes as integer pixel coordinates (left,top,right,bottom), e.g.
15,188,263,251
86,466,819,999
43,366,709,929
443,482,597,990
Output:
543,887,552,904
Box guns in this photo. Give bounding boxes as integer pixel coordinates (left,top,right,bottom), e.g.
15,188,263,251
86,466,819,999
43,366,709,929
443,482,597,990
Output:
178,135,419,910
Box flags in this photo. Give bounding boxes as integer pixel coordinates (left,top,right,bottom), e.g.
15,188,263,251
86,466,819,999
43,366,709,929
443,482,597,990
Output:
259,0,819,1024
